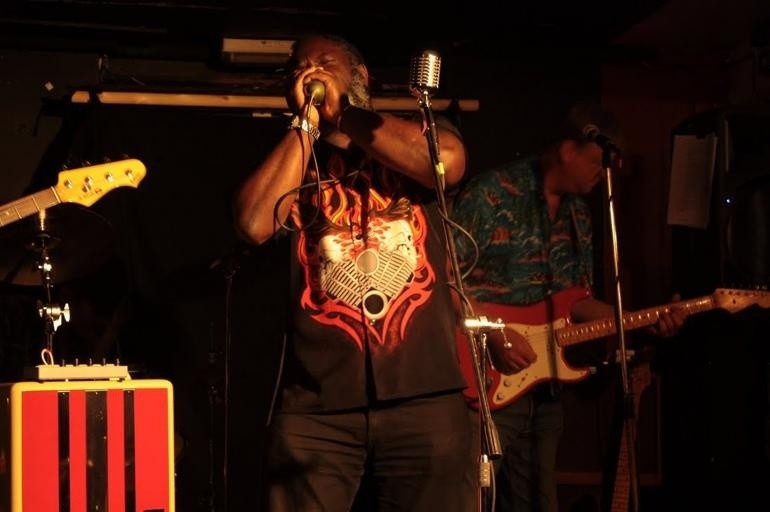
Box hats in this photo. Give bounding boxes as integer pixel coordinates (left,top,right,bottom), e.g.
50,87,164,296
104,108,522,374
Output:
560,102,626,154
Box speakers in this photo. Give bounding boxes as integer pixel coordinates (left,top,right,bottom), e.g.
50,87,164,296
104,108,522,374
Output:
663,318,770,490
672,105,770,317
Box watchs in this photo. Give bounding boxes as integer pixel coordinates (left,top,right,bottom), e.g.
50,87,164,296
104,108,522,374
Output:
287,114,321,142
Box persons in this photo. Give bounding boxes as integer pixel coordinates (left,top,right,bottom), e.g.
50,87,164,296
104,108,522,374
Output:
444,100,686,511
230,26,483,512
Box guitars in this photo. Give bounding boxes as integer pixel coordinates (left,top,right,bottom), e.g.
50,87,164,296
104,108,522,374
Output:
0,156,146,230
470,286,769,410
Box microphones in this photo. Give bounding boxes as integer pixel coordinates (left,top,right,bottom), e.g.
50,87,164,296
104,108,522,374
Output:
305,80,328,107
582,122,625,161
32,208,54,231
408,47,442,98
